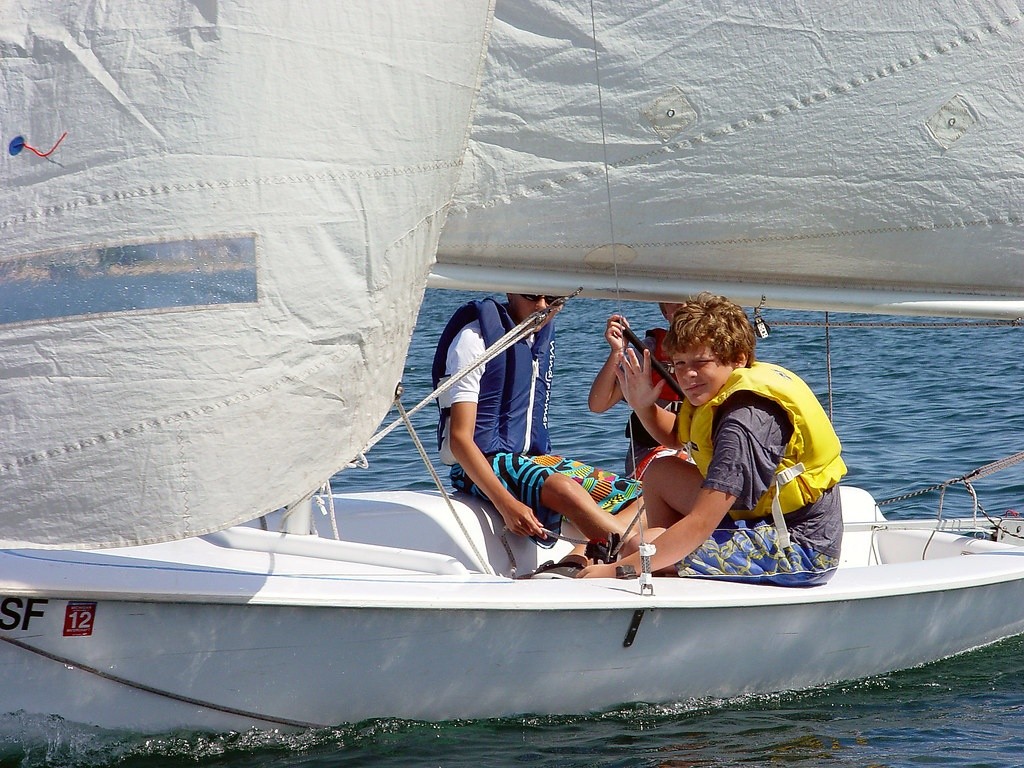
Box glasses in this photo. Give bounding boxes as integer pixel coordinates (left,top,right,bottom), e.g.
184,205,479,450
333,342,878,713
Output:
520,294,565,306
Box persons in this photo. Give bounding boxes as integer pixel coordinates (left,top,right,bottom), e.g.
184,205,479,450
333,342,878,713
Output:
587,302,695,483
577,292,847,586
432,294,648,580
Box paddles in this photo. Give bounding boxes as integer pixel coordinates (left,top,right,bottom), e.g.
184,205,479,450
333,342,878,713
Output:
613,310,694,403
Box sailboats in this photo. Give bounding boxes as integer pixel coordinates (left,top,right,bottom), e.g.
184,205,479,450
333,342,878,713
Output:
0,0,1023,740
